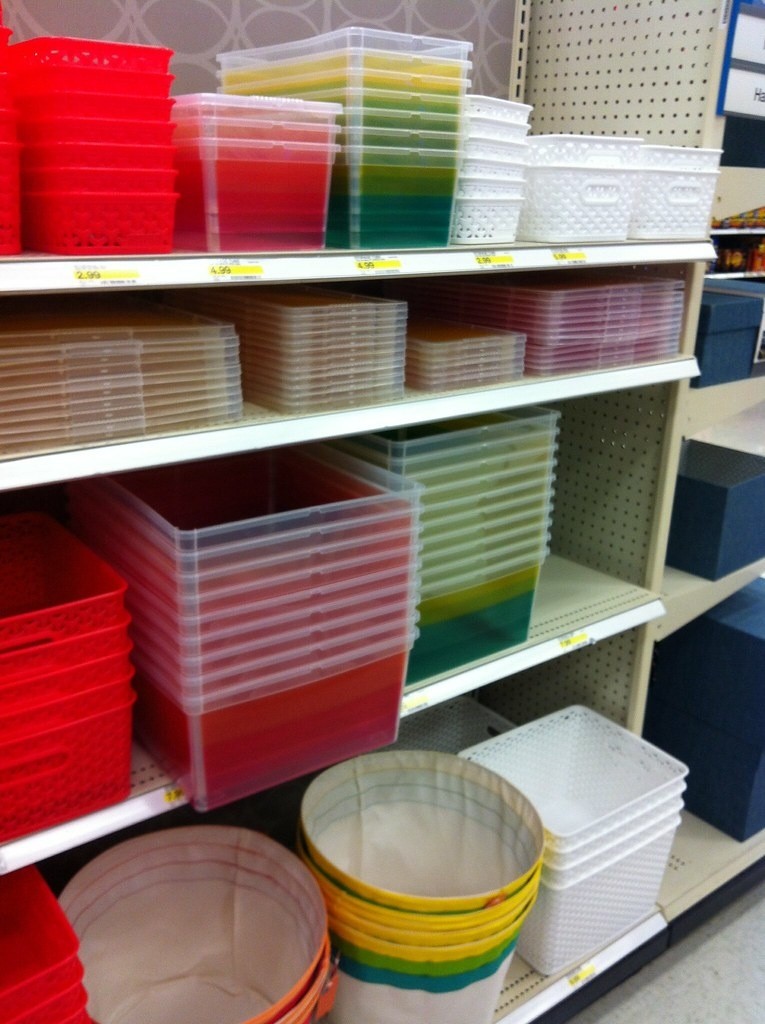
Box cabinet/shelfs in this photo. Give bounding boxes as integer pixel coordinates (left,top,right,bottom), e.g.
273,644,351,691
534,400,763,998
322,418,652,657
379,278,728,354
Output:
654,374,765,950
0,4,732,1024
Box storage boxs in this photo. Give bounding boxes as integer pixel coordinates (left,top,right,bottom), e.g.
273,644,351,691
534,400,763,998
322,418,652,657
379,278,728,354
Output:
1,2,732,1023
689,277,764,389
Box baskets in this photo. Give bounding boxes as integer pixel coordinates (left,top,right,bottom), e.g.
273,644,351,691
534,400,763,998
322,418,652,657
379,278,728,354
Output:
449,93,723,245
0,514,139,842
457,703,689,977
0,3,184,256
0,864,97,1024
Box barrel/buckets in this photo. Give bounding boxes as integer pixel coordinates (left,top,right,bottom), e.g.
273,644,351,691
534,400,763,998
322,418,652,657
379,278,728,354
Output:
55,825,333,1024
295,750,546,1024
